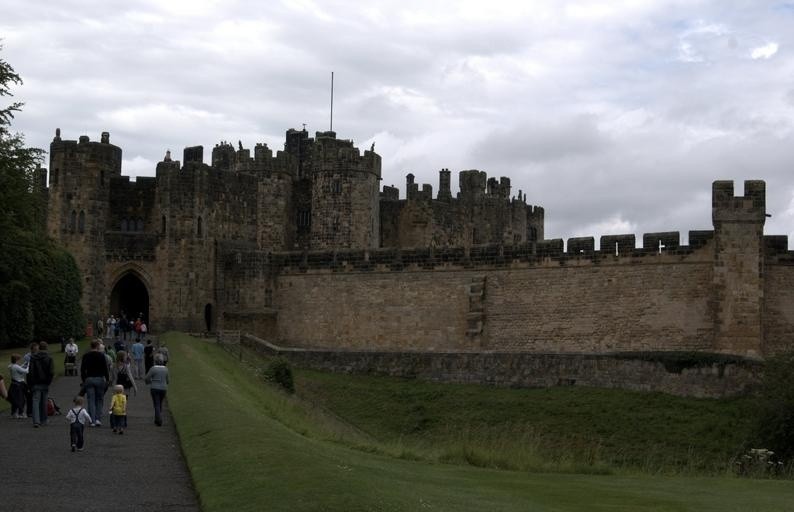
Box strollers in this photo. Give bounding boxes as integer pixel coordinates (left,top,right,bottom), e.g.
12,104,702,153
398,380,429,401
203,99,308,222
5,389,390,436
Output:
64,351,78,376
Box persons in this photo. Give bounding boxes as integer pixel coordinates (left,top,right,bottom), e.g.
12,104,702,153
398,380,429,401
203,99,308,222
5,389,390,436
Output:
0,311,169,452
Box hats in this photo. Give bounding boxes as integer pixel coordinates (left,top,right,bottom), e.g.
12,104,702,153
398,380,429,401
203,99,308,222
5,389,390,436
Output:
39,342,48,351
113,385,123,391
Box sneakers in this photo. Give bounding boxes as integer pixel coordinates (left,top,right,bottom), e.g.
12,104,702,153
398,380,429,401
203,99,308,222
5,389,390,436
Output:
135,375,143,379
112,427,124,434
91,419,104,427
71,445,84,452
12,412,27,418
34,419,50,428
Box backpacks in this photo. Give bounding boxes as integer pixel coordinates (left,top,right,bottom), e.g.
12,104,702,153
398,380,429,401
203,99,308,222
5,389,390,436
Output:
48,398,56,415
